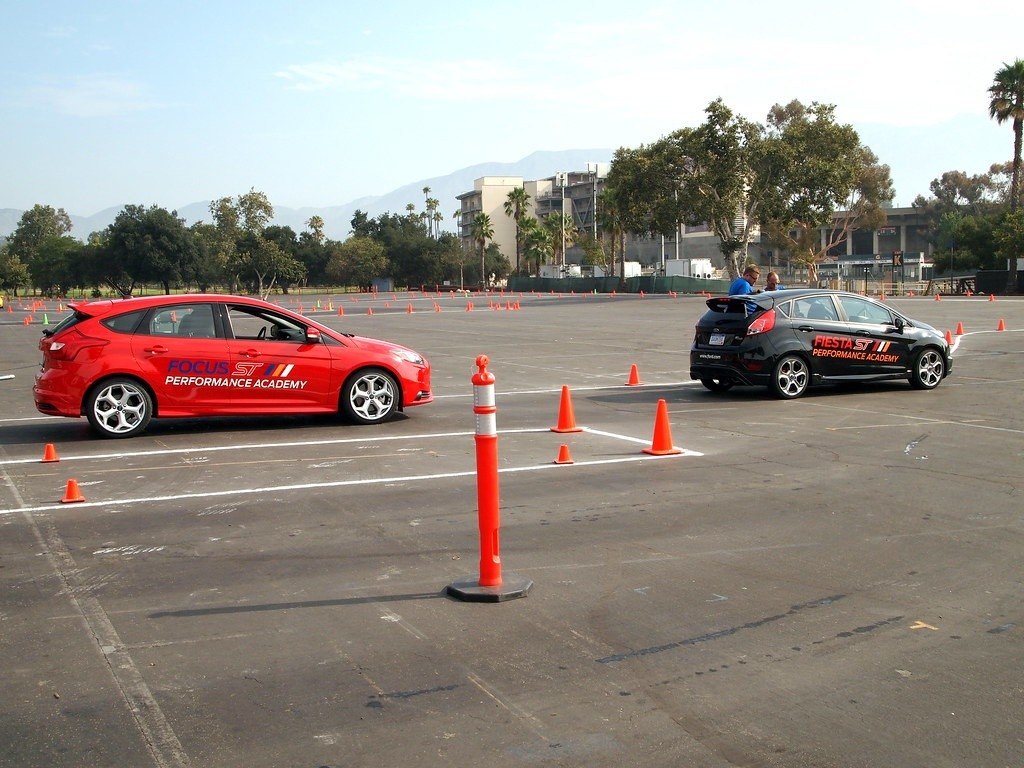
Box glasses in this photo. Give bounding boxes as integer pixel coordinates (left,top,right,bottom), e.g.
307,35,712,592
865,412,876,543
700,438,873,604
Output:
749,275,757,280
768,280,780,285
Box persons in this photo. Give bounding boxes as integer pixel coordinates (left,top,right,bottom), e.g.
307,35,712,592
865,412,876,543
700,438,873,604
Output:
729,264,759,296
764,272,786,291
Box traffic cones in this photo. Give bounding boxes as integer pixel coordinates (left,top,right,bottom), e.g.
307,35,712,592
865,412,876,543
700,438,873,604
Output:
880,290,1005,345
554,445,574,464
550,385,583,433
641,399,683,456
41,444,60,463
625,364,644,386
0,294,91,327
275,288,711,317
61,478,85,503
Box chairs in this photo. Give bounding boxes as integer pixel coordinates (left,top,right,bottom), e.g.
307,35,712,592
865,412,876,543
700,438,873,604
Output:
177,314,191,335
807,303,826,319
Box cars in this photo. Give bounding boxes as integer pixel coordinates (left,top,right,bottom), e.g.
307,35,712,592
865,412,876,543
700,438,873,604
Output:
690,288,954,399
32,293,433,439
